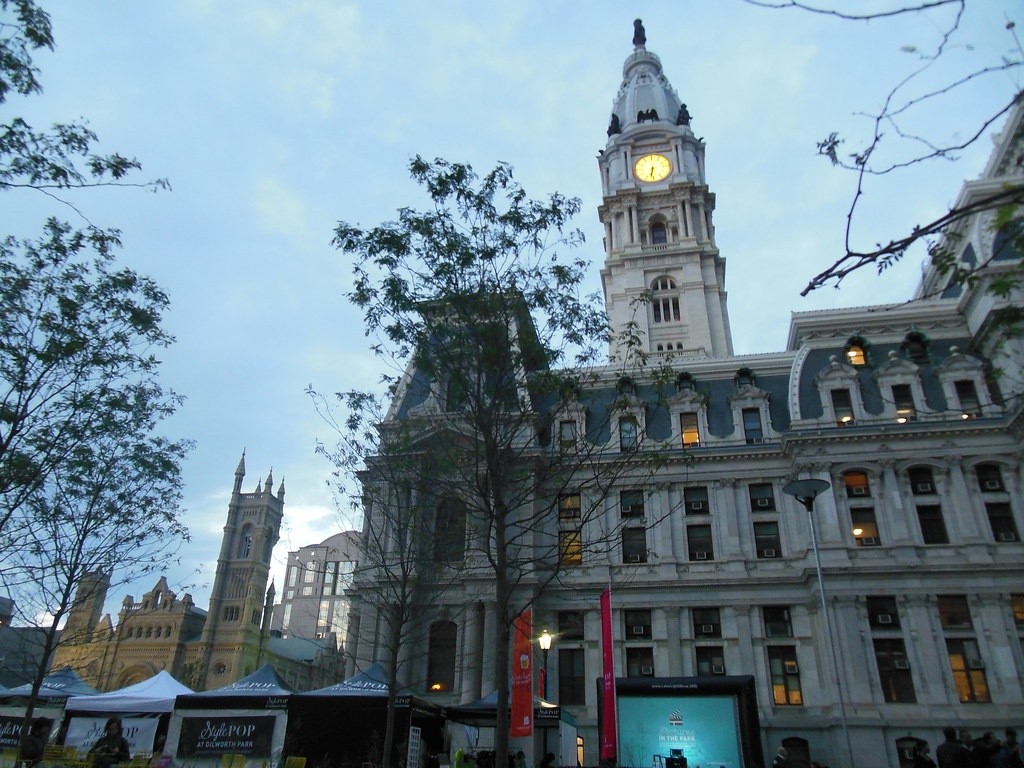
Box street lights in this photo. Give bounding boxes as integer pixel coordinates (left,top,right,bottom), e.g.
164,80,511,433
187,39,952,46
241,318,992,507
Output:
782,477,860,768
539,629,553,764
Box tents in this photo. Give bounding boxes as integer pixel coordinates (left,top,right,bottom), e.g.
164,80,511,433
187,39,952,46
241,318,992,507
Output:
0,664,100,746
14,717,51,768
57,672,195,759
281,663,447,768
446,677,577,768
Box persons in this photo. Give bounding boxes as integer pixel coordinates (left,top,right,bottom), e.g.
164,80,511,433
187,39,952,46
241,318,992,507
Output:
913,740,937,768
607,114,619,136
163,663,300,768
539,753,555,768
677,104,692,125
508,750,527,768
453,749,496,768
87,717,130,768
773,747,821,768
936,727,1024,768
424,748,440,768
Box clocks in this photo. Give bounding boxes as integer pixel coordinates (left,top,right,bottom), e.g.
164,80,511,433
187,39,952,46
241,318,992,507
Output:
633,153,674,184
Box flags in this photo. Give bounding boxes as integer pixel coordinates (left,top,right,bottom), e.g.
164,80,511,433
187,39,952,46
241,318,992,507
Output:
510,608,533,737
600,585,617,759
